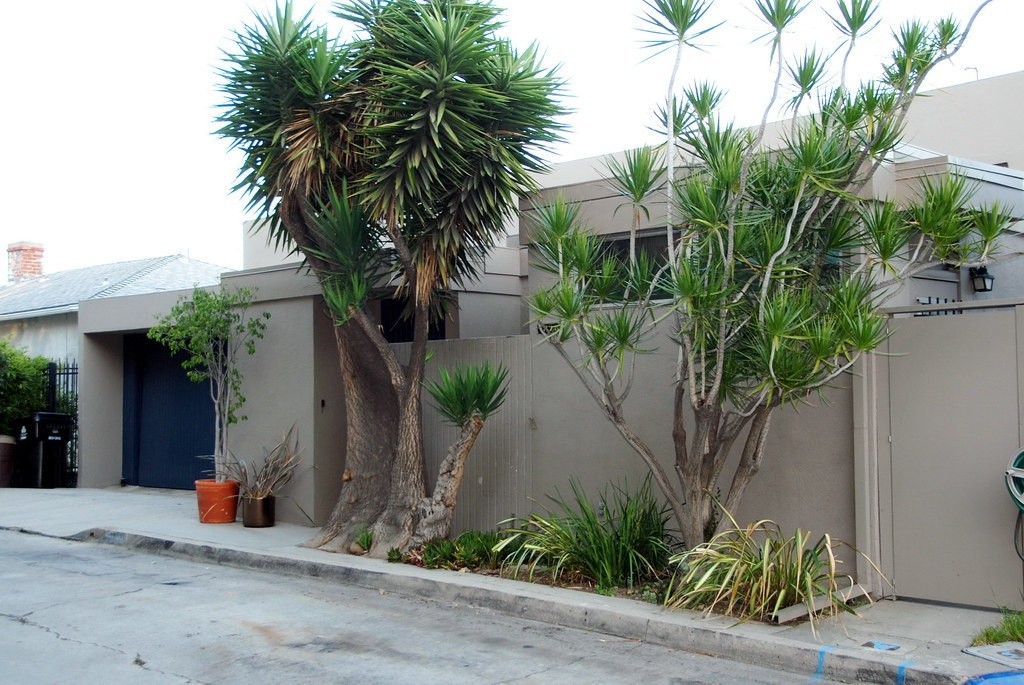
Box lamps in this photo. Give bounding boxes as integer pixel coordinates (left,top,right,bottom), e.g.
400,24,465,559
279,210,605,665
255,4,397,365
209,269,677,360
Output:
968,264,995,292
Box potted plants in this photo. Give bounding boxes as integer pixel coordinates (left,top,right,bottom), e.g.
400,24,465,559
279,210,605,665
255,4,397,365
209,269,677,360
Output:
146,281,305,527
